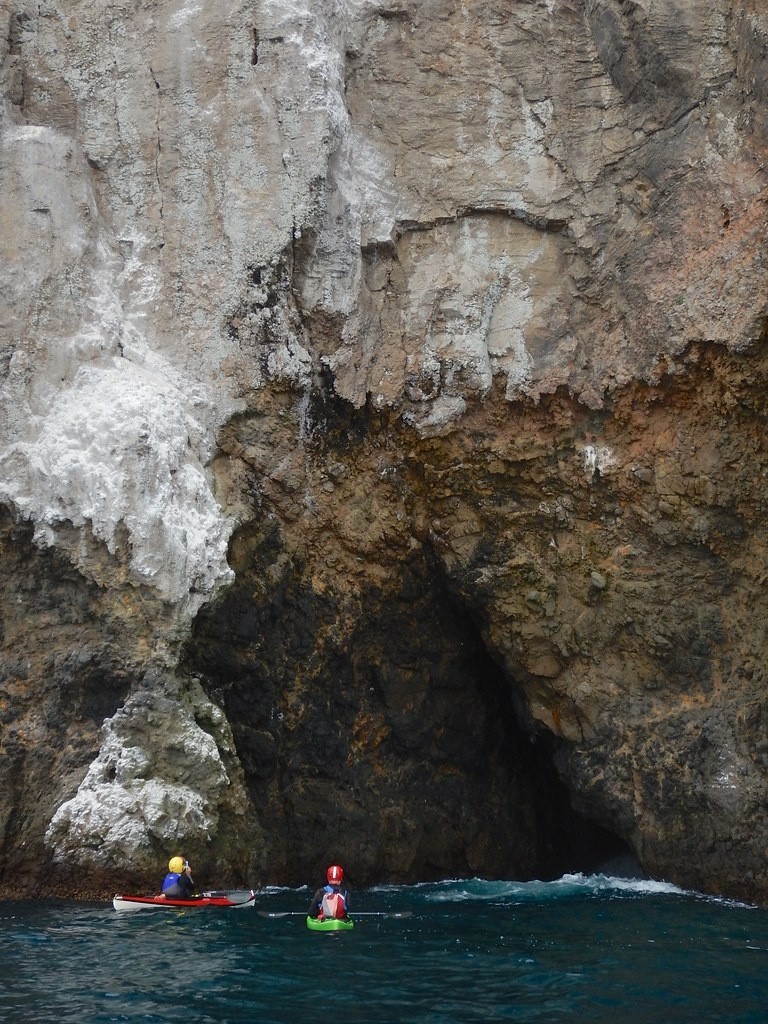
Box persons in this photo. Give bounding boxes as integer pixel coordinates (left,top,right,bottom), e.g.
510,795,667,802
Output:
162,857,195,898
307,866,351,923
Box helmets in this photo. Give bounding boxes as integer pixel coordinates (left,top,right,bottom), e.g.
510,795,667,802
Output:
168,856,187,873
328,866,343,884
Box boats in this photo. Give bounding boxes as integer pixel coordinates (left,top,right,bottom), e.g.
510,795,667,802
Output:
110,887,257,912
305,910,355,933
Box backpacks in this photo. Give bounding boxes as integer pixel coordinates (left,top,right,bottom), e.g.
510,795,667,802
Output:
322,892,345,919
